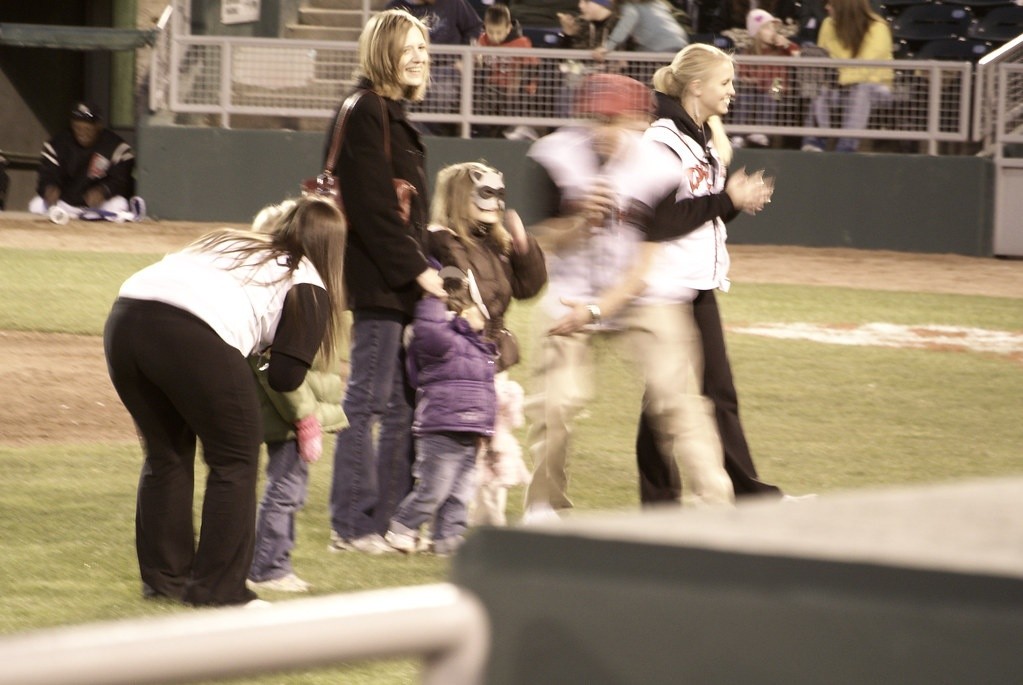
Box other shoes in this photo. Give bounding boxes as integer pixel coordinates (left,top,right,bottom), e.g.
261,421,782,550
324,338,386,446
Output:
247,573,311,593
747,133,769,147
731,136,746,146
801,143,822,152
386,530,429,553
432,535,465,557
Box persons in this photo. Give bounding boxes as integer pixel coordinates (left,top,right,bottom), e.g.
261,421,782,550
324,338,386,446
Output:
386,0,486,139
522,64,737,522
35,100,138,209
104,201,347,611
799,0,892,151
324,9,434,557
383,258,501,553
636,44,801,509
546,0,686,116
428,159,549,529
729,7,799,150
458,4,537,135
245,200,351,593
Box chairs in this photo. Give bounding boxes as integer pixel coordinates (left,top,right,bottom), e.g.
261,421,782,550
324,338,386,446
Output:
473,0,1023,158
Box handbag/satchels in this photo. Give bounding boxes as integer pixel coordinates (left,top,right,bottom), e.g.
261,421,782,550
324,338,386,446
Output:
301,90,416,224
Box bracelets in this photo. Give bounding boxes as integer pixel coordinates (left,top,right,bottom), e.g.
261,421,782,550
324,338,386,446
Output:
786,42,798,54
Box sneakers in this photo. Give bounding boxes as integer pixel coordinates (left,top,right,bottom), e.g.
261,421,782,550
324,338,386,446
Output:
327,530,397,555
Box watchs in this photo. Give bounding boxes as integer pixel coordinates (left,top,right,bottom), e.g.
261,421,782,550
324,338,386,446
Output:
586,304,601,324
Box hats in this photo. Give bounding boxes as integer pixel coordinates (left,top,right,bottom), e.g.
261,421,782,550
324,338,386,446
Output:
745,9,783,37
69,103,100,124
